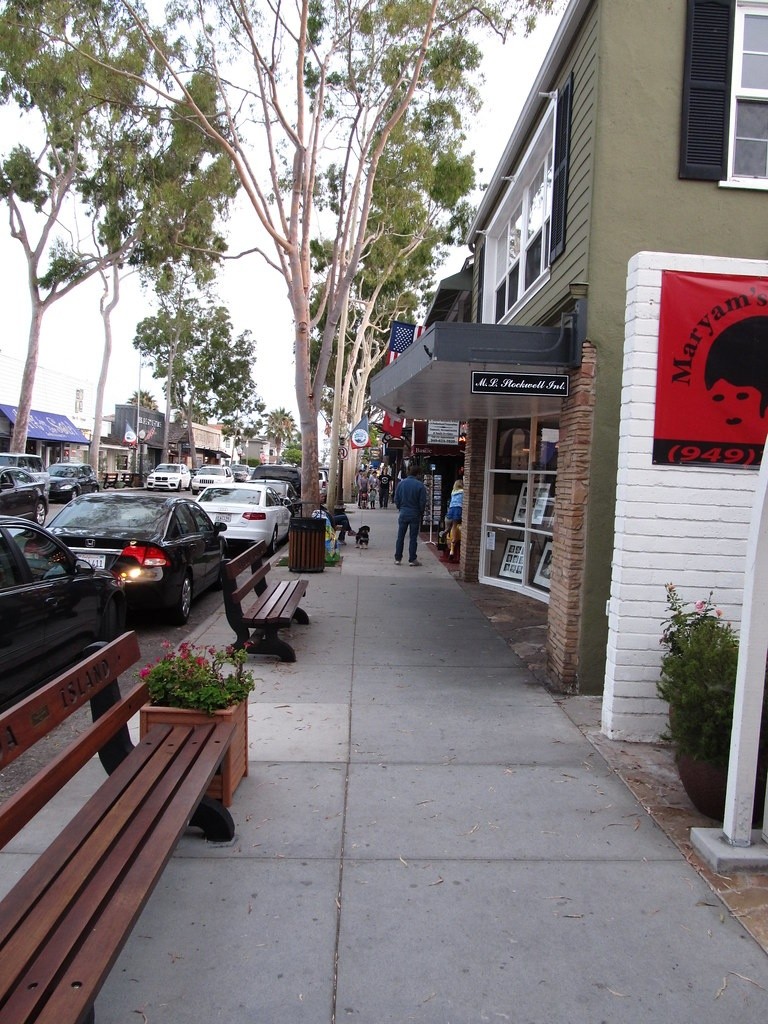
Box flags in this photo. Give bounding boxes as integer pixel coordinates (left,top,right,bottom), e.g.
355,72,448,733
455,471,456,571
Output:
350,413,371,449
385,322,426,366
324,421,332,438
122,423,137,446
382,410,404,438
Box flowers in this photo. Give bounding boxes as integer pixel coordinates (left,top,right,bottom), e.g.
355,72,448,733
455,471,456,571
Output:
136,641,255,717
655,581,742,764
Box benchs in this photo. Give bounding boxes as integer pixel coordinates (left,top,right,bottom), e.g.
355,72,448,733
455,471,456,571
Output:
121,473,134,488
0,630,238,1024
220,540,310,661
103,473,118,489
323,503,347,530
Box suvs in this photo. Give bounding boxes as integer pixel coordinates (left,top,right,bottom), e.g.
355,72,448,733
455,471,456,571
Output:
146,462,191,492
191,465,236,495
250,461,302,507
0,451,51,502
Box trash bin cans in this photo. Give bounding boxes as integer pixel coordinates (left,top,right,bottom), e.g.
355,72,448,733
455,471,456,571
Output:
288,501,328,572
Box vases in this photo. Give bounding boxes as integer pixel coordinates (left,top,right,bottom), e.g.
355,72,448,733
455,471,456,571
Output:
670,703,768,826
140,695,249,805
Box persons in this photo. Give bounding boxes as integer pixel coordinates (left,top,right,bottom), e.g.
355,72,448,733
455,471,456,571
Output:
518,487,547,520
504,546,524,575
394,465,427,566
320,492,357,545
542,553,552,578
356,469,378,509
441,480,463,561
378,469,393,509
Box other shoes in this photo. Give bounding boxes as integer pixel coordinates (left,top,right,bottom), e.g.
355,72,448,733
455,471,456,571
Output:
450,552,454,561
348,531,357,536
338,541,347,545
395,560,401,565
371,508,374,509
380,505,383,508
373,508,376,510
440,531,445,538
409,559,423,566
384,507,388,510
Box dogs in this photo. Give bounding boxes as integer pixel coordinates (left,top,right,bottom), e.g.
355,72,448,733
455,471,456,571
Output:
355,525,370,549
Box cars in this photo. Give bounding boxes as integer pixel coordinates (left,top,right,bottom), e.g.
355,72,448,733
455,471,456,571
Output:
43,488,229,625
188,463,339,504
189,482,294,556
47,462,100,504
0,513,128,716
244,478,299,519
0,466,50,526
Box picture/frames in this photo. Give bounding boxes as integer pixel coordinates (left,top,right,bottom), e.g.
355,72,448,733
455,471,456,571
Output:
530,538,553,593
498,538,535,582
511,481,553,526
